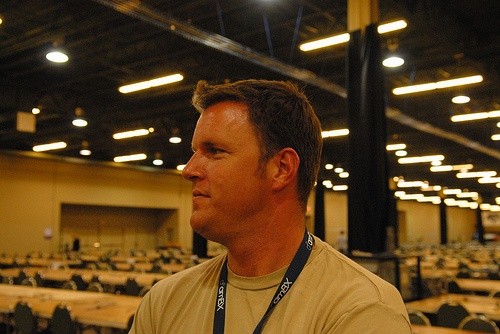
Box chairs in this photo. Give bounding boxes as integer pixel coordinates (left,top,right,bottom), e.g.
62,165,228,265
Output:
21,277,38,287
408,312,432,327
7,302,38,334
85,282,103,292
60,280,78,290
458,314,500,334
448,280,464,294
47,304,77,334
437,302,470,328
125,278,141,296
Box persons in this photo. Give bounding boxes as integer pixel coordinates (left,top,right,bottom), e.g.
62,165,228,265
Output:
127,78,413,334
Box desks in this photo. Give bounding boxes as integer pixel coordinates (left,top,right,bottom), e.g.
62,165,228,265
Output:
0,246,218,334
347,240,500,334
